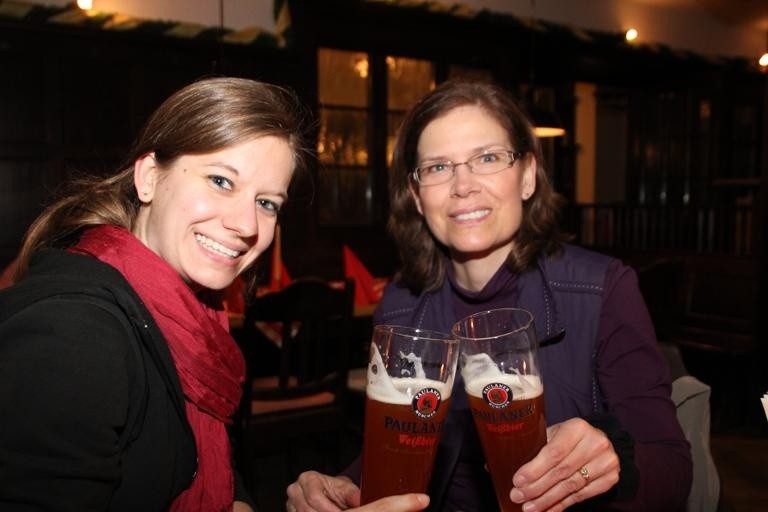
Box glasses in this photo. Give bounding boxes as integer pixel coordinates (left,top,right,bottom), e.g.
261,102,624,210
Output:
413,150,520,186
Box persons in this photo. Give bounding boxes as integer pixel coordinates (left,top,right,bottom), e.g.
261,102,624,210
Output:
285,71,692,512
0,75,431,512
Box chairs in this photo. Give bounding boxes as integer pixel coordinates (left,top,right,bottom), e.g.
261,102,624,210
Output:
231,277,355,493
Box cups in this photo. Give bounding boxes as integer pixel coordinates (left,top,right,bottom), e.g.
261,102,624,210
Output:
451,308,548,512
360,323,461,504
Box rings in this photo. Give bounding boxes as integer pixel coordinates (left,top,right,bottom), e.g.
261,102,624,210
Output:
578,468,591,485
